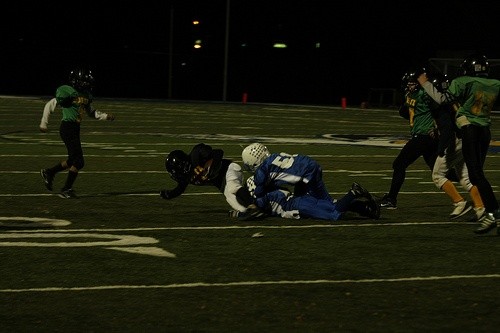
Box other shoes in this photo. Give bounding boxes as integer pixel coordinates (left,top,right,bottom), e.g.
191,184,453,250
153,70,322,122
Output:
448,200,472,220
362,198,380,220
40,168,54,191
474,213,497,235
374,195,398,210
351,181,372,200
56,188,73,199
465,207,487,223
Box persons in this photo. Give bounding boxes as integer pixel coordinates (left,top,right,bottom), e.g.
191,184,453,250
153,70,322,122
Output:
245,174,372,222
376,51,500,233
242,142,381,222
159,142,249,213
38,63,116,200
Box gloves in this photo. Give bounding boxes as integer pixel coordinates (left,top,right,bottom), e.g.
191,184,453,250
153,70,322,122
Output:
228,210,239,218
159,190,169,200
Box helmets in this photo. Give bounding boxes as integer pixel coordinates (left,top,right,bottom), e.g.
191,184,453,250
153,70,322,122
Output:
458,52,491,78
246,176,257,195
429,72,450,92
242,143,271,167
401,69,422,88
69,66,93,82
165,150,189,174
